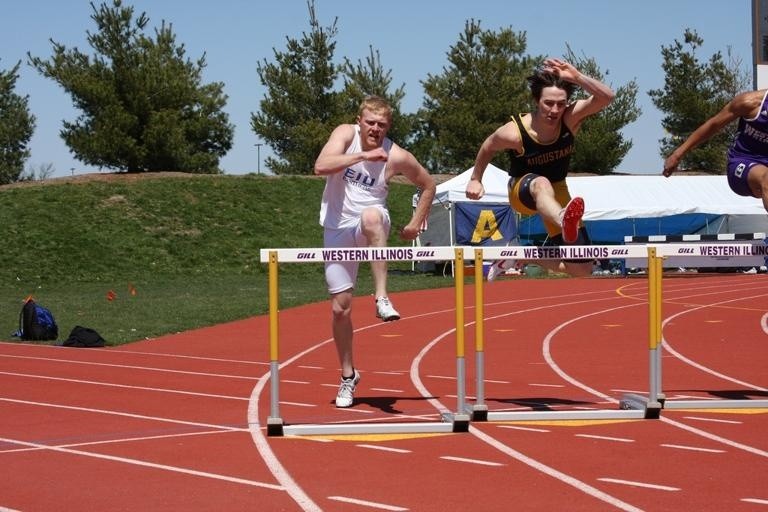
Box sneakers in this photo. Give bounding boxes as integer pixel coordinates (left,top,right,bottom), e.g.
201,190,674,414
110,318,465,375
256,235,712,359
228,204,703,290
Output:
560,197,584,243
487,259,516,281
335,367,360,407
375,296,400,322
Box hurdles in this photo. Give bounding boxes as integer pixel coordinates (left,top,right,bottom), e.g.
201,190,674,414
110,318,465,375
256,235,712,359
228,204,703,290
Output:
258,242,768,438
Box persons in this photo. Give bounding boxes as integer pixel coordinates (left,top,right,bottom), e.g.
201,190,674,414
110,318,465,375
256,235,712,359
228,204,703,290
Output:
467,58,616,281
662,87,768,212
314,94,436,409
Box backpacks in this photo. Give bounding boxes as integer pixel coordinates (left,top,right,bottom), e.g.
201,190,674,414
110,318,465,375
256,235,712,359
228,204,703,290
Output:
19,300,58,341
